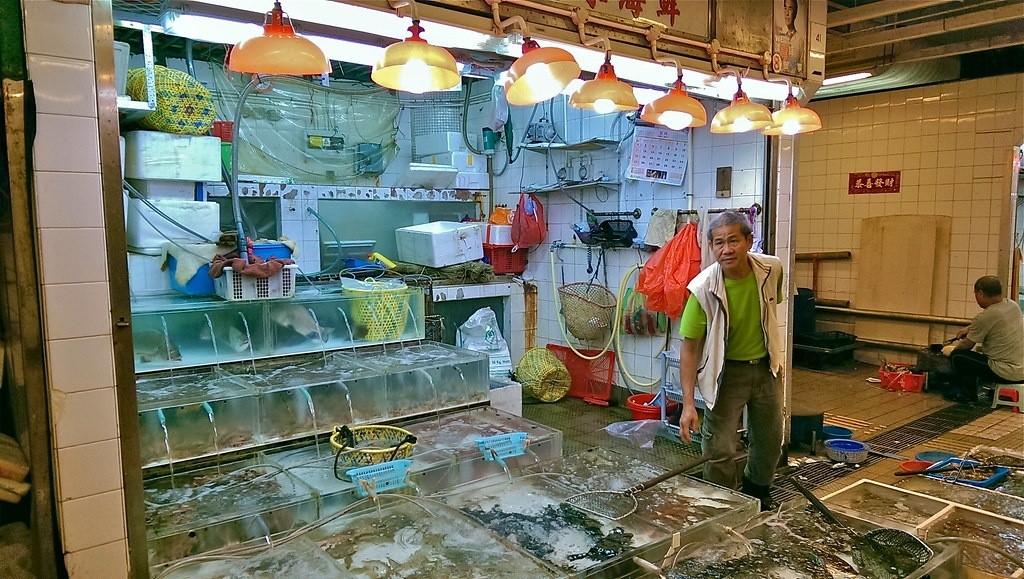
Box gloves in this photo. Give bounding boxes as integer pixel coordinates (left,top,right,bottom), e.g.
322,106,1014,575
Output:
941,346,956,356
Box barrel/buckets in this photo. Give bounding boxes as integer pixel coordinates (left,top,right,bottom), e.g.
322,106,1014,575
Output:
251,243,291,261
162,242,214,294
627,394,677,421
220,142,231,179
793,288,815,344
210,121,234,142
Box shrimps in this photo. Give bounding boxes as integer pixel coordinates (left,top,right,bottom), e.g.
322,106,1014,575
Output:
138,383,487,469
324,516,513,579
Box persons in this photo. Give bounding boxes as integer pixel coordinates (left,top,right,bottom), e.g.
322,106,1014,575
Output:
780,0,804,72
679,212,785,511
943,276,1024,402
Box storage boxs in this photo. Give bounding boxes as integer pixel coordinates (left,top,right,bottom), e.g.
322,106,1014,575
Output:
799,330,859,367
395,130,528,275
879,362,926,393
118,130,563,569
926,457,1010,491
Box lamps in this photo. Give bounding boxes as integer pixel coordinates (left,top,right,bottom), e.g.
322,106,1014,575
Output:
504,36,580,106
568,58,640,115
710,85,773,134
761,93,823,136
371,19,460,94
639,75,707,130
229,0,332,76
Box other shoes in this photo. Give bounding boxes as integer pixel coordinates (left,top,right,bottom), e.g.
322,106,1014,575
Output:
742,476,780,511
942,393,978,404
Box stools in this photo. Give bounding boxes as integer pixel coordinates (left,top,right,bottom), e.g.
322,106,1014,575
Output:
990,383,1024,414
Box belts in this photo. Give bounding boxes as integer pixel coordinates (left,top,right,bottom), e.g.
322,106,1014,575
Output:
730,356,767,364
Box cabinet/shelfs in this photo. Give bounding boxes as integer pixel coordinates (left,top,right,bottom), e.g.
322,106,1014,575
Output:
661,350,707,445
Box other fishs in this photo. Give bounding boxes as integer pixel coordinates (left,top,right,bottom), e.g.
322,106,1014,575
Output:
458,499,636,563
200,322,248,353
271,304,335,343
133,329,183,364
694,539,860,579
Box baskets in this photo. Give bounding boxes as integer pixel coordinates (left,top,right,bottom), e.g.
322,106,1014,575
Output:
330,424,417,466
824,440,869,463
512,347,571,402
209,262,298,301
598,219,638,247
341,282,410,341
125,66,216,135
558,281,616,340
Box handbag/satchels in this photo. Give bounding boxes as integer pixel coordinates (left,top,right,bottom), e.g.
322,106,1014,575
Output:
510,193,546,252
619,288,665,337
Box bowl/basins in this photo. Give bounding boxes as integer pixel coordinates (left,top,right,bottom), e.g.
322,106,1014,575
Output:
899,450,958,474
823,426,851,440
824,438,870,463
